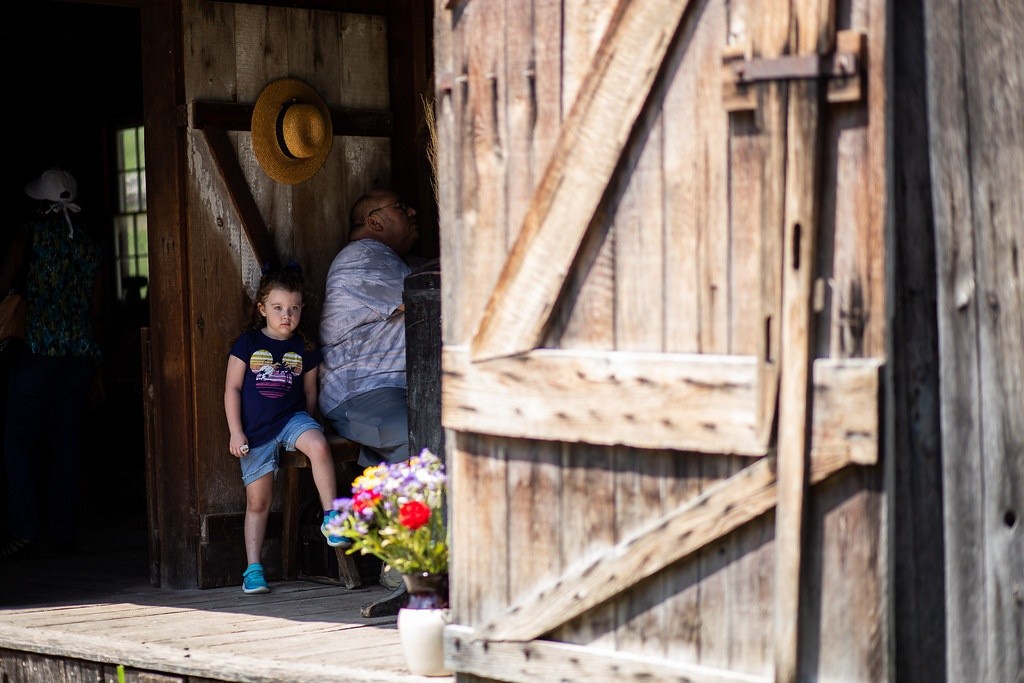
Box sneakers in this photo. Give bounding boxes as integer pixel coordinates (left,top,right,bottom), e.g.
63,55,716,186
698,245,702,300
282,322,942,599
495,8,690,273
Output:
379,560,403,590
242,563,270,594
321,511,354,547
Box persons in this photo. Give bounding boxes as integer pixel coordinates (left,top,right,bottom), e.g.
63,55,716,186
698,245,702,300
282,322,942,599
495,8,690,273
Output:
1,167,103,561
318,193,420,462
222,261,355,594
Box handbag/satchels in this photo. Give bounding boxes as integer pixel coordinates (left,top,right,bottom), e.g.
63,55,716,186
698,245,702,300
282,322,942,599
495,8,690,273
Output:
0,288,27,365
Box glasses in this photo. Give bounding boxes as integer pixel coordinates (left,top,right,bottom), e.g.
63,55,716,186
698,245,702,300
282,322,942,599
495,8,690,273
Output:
368,199,407,215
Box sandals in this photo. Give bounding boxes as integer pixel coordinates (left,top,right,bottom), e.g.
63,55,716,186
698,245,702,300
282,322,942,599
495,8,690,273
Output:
3,537,42,561
50,539,78,552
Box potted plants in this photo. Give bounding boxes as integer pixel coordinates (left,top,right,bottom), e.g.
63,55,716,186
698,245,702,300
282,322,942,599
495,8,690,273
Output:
392,562,458,680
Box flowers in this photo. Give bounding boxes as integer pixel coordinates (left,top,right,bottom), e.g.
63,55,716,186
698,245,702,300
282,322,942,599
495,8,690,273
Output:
322,450,457,589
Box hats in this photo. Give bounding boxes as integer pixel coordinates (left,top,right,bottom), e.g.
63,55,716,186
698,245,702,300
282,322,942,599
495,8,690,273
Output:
251,78,333,184
25,168,77,202
